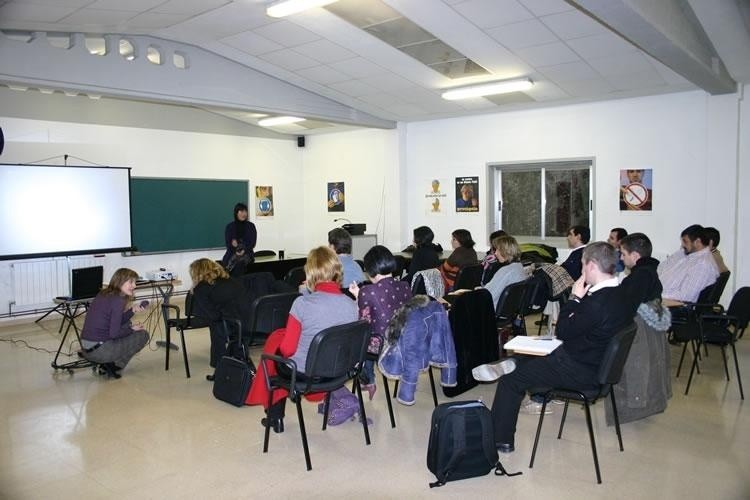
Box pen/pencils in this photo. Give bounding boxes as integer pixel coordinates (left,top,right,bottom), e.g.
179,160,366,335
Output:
534,339,552,340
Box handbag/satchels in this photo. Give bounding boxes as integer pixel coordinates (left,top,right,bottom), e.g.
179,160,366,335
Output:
317,382,365,427
212,339,256,408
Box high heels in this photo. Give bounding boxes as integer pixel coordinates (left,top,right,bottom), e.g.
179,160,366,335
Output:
360,382,376,401
91,361,123,379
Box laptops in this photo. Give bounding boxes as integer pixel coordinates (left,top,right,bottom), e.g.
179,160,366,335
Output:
55,265,103,300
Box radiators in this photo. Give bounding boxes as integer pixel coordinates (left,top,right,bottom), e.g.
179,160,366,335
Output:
13,254,97,308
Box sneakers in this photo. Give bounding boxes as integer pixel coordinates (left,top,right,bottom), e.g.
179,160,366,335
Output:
552,399,564,406
519,400,555,415
535,317,548,325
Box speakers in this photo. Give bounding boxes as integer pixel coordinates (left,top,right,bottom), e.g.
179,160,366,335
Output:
298,137,305,147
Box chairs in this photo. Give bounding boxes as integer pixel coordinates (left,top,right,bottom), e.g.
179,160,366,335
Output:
162,248,731,377
261,319,370,472
528,322,639,484
213,293,305,407
352,296,439,428
673,285,750,400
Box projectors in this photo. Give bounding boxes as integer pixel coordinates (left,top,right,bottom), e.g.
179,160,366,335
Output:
145,270,177,281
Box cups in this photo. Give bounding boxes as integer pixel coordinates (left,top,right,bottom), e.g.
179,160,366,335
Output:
278,251,284,259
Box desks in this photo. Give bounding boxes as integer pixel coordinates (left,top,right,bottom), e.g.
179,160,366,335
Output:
51,278,183,376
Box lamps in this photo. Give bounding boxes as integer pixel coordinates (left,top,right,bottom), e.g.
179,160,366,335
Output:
0,29,186,69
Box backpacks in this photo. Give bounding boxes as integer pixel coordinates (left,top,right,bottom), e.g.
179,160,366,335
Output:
426,400,499,484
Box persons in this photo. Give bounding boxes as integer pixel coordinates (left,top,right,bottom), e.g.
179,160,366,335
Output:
80,268,149,378
456,185,478,209
620,170,652,210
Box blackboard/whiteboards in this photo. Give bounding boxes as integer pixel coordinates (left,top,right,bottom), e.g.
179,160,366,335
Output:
122,178,251,257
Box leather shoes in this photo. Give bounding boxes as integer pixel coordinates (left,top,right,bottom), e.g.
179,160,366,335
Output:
206,375,215,382
471,357,517,382
496,440,515,453
261,416,284,433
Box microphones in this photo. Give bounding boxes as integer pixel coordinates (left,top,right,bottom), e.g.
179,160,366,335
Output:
334,218,352,224
